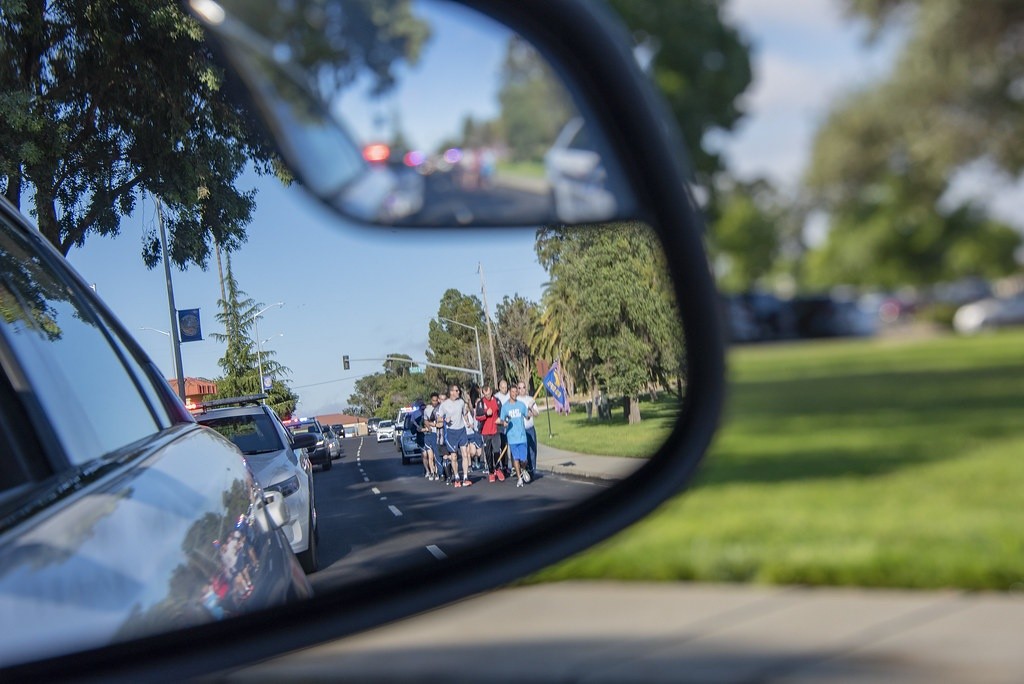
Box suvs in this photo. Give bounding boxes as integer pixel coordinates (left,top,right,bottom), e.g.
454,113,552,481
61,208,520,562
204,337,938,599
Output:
189,392,316,575
284,417,333,470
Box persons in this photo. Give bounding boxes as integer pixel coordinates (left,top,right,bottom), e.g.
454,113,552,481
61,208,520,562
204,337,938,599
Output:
410,381,539,489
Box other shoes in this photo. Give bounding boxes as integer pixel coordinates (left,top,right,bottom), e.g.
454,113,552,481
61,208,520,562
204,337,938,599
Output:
516,478,523,488
521,469,530,483
455,479,461,488
425,472,460,485
468,460,489,475
510,467,517,477
462,478,472,486
496,470,505,482
489,473,496,483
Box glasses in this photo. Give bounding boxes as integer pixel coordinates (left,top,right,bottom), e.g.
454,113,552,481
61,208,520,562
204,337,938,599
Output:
450,390,460,392
519,387,526,390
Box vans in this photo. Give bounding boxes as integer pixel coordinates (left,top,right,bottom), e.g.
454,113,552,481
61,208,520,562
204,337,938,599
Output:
392,408,418,447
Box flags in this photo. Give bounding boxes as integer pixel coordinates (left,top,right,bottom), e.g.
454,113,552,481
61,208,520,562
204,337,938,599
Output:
543,361,570,416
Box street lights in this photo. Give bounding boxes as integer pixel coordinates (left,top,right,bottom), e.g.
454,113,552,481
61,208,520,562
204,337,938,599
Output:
139,326,190,407
254,301,288,406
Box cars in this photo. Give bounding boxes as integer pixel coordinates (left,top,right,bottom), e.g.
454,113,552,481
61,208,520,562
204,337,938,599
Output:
396,411,423,465
1,193,319,674
322,424,341,460
734,275,916,343
330,423,346,438
284,419,328,470
368,418,384,434
377,420,396,443
